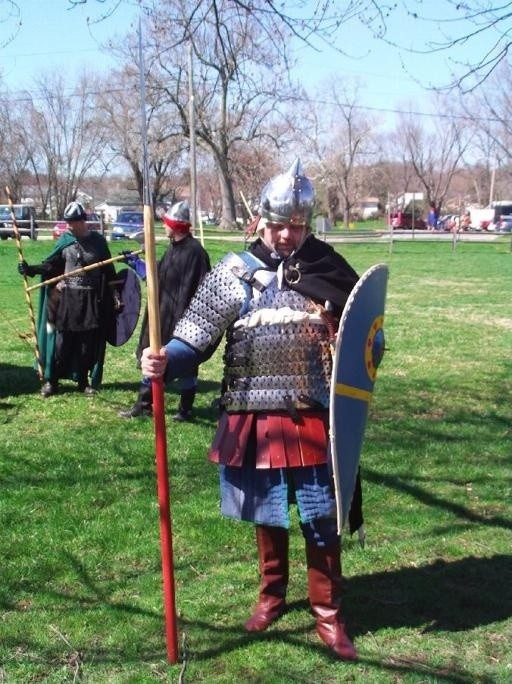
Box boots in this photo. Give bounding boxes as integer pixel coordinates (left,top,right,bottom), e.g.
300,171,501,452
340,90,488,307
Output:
243,526,288,631
307,548,358,662
172,394,198,421
121,384,153,418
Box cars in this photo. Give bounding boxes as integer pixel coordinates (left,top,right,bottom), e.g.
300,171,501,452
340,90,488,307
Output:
498,214,512,233
53,210,108,245
387,211,427,230
112,209,145,244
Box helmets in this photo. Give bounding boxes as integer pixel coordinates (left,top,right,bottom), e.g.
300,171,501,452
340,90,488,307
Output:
162,201,191,233
259,158,317,226
63,202,87,220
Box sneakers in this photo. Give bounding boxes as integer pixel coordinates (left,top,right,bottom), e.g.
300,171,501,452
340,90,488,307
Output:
77,384,99,394
41,382,58,396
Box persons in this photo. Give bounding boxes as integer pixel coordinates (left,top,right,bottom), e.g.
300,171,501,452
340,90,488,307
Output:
460,211,471,231
114,198,211,422
139,157,388,663
17,203,124,397
426,201,437,234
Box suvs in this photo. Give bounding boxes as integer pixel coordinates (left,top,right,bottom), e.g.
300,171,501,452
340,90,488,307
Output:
0,204,39,241
